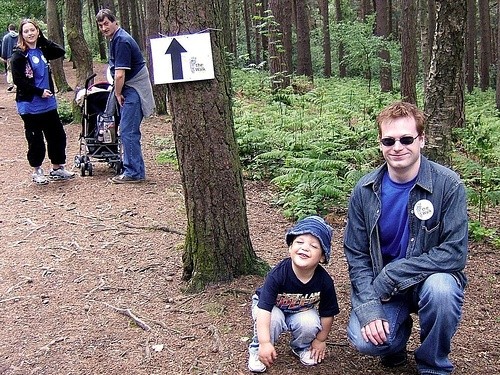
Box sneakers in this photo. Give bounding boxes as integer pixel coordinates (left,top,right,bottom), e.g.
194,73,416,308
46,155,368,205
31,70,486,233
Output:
247,347,266,371
109,173,140,184
6,87,13,92
381,315,414,366
293,349,316,366
33,170,49,184
51,166,76,180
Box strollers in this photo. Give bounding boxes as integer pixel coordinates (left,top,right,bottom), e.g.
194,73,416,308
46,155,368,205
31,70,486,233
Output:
74,74,124,177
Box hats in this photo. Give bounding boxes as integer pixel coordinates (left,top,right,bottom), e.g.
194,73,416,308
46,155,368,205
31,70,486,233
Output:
285,216,333,263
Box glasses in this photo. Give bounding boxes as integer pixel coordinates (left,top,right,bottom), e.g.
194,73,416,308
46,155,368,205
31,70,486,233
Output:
380,134,418,144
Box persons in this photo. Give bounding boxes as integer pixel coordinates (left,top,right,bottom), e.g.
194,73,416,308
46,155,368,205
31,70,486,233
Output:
344,103,470,375
248,216,340,372
0,19,77,185
94,9,155,183
